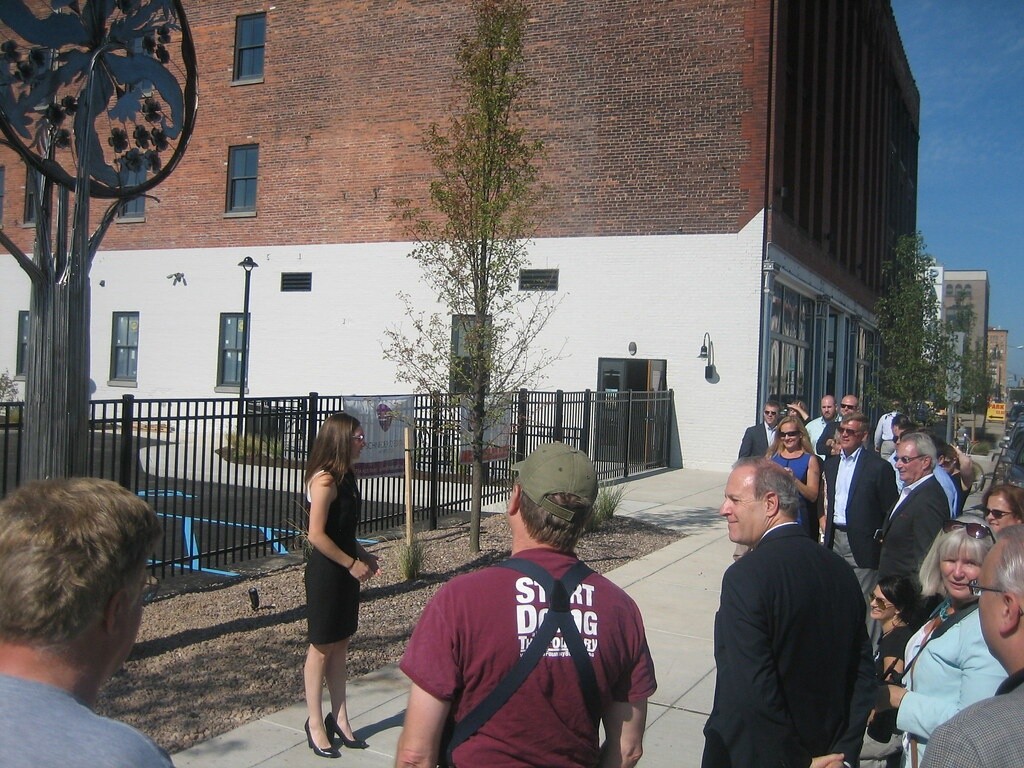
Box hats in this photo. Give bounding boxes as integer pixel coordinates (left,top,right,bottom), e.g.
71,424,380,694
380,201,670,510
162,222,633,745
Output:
510,442,598,521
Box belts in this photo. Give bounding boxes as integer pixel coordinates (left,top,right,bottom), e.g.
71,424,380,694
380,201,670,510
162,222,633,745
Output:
833,523,847,532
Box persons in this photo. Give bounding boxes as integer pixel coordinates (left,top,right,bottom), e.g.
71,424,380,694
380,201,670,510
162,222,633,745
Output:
701,455,879,768
736,394,976,633
394,443,658,768
302,412,378,758
859,485,1024,768
0,477,175,768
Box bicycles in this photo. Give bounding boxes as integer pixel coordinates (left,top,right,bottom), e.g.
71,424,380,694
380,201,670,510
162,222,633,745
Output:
953,433,986,495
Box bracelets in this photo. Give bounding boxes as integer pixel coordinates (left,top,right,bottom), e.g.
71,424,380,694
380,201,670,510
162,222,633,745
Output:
346,558,357,570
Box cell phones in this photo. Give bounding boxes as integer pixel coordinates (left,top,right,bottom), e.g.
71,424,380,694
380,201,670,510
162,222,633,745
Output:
874,528,882,540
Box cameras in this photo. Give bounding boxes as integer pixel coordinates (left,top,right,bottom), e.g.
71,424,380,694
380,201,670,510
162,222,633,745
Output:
867,681,907,744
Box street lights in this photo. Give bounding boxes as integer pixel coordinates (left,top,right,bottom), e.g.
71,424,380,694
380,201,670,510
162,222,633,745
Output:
236,255,260,438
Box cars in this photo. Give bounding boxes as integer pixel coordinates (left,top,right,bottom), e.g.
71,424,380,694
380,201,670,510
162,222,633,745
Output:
989,385,1024,495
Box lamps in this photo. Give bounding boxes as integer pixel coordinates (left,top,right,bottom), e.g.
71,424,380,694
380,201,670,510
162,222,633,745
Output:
168,272,185,282
697,332,714,378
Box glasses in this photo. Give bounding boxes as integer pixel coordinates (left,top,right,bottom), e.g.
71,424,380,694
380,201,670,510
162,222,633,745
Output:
765,410,778,416
353,434,365,443
778,431,801,437
892,435,899,443
894,455,924,463
982,507,1018,520
840,403,857,410
869,592,896,611
837,426,861,435
942,518,996,546
967,579,1024,617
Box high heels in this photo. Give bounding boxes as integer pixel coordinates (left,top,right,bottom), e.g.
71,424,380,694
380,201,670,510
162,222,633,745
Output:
325,712,369,749
305,716,343,758
